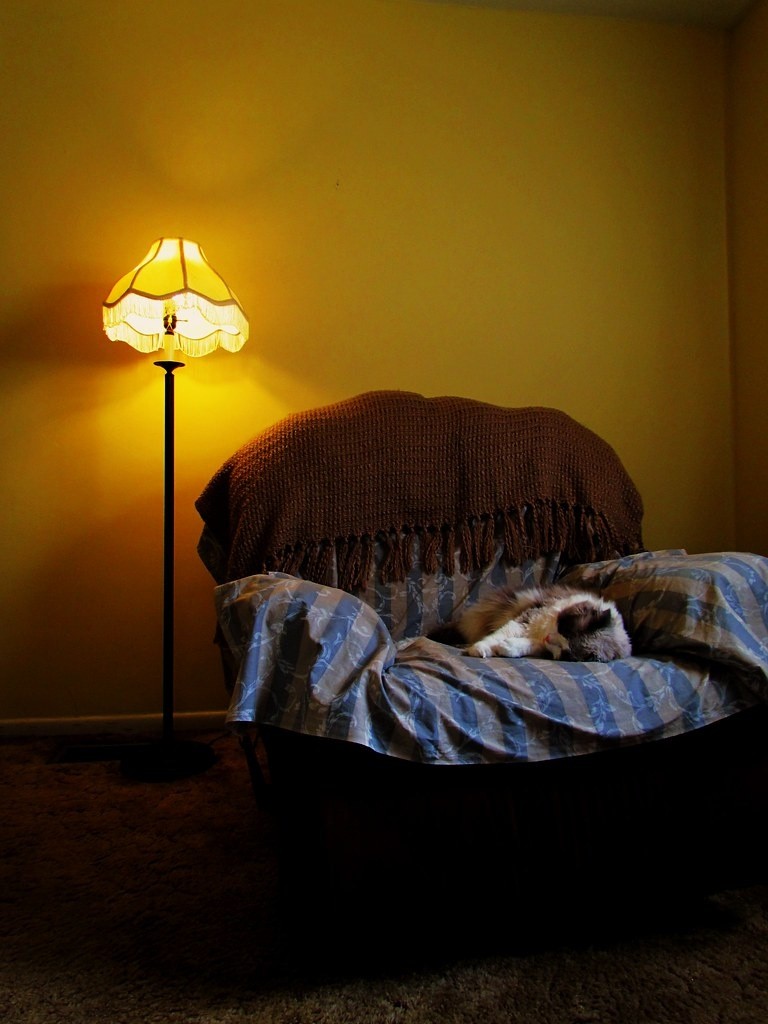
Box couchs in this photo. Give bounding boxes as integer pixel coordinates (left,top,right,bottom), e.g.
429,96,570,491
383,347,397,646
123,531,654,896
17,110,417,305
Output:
196,389,767,914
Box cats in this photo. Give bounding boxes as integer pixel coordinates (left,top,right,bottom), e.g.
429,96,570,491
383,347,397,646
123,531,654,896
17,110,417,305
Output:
426,582,632,663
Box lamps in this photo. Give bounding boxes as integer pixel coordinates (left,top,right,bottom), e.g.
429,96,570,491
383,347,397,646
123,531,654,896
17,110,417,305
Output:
96,238,252,787
